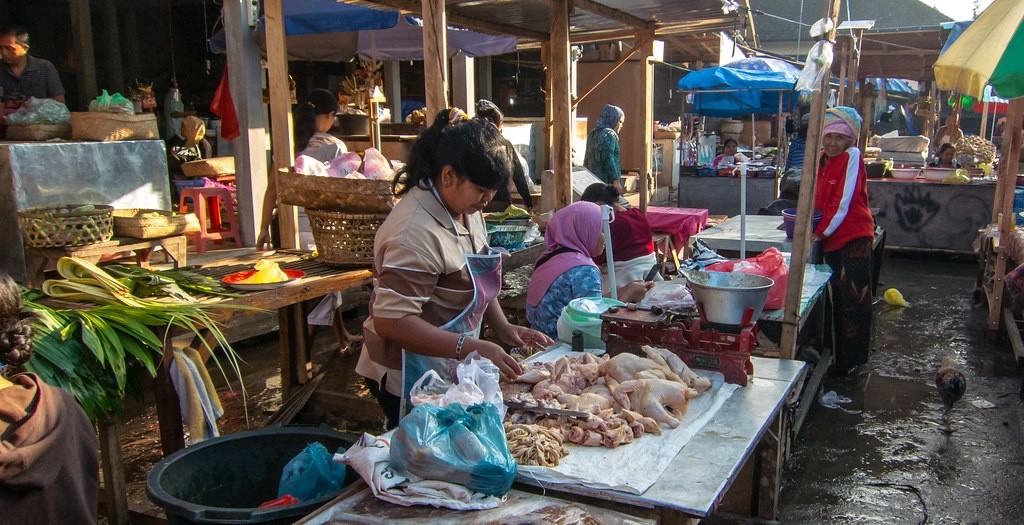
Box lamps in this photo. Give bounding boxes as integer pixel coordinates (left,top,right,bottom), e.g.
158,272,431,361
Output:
166,4,180,102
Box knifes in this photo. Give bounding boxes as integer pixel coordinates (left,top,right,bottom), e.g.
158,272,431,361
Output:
505,402,589,419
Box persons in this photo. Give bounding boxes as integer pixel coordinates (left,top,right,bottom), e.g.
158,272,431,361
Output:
583,104,625,194
179,116,212,159
355,107,555,428
0,24,66,105
927,143,962,169
257,88,348,250
165,81,196,146
933,113,963,149
873,112,893,137
713,138,744,167
810,106,874,365
581,183,655,294
785,113,810,172
526,201,655,342
908,94,931,134
474,101,536,214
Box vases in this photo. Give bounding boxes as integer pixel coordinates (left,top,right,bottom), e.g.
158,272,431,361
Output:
336,114,370,136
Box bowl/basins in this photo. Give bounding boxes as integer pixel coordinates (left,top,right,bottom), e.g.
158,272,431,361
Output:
889,169,919,179
866,163,887,178
782,209,822,237
492,224,529,247
486,224,496,245
923,168,955,179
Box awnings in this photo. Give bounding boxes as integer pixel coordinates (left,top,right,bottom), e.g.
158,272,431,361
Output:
932,0,1024,104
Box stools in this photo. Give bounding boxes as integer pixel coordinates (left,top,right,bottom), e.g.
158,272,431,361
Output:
179,186,242,253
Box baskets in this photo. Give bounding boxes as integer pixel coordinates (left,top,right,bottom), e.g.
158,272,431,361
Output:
111,207,187,239
278,164,405,264
16,203,114,247
70,110,159,141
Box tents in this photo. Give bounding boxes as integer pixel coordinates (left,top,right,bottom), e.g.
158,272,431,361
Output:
210,0,517,106
678,57,802,160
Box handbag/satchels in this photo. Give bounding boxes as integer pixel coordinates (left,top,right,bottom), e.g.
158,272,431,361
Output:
388,349,518,494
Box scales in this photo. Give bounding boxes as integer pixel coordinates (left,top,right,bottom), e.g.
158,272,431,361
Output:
600,266,777,387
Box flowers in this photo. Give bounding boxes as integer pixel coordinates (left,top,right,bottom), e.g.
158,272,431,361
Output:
326,55,387,112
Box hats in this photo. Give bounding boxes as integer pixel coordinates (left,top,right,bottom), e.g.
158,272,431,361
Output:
822,105,862,146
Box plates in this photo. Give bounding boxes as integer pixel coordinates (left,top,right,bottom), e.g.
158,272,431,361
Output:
222,270,305,290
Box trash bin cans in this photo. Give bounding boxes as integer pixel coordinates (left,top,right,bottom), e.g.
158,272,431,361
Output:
145,424,362,525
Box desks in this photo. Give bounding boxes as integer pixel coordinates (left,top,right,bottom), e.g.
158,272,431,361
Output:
0,115,885,525
680,177,998,253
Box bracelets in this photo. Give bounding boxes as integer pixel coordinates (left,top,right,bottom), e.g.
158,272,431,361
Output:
456,334,468,361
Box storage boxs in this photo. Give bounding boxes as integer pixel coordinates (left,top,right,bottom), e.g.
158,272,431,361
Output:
598,42,623,61
881,135,929,151
662,415,778,525
172,179,214,202
974,102,1009,113
696,156,777,178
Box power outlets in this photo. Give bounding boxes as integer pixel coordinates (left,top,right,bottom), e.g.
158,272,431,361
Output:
248,0,260,26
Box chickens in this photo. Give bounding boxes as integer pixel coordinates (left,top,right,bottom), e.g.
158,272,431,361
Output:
934,355,967,424
499,342,712,466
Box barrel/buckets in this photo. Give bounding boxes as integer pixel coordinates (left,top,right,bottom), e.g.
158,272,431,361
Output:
336,114,369,136
147,427,362,525
